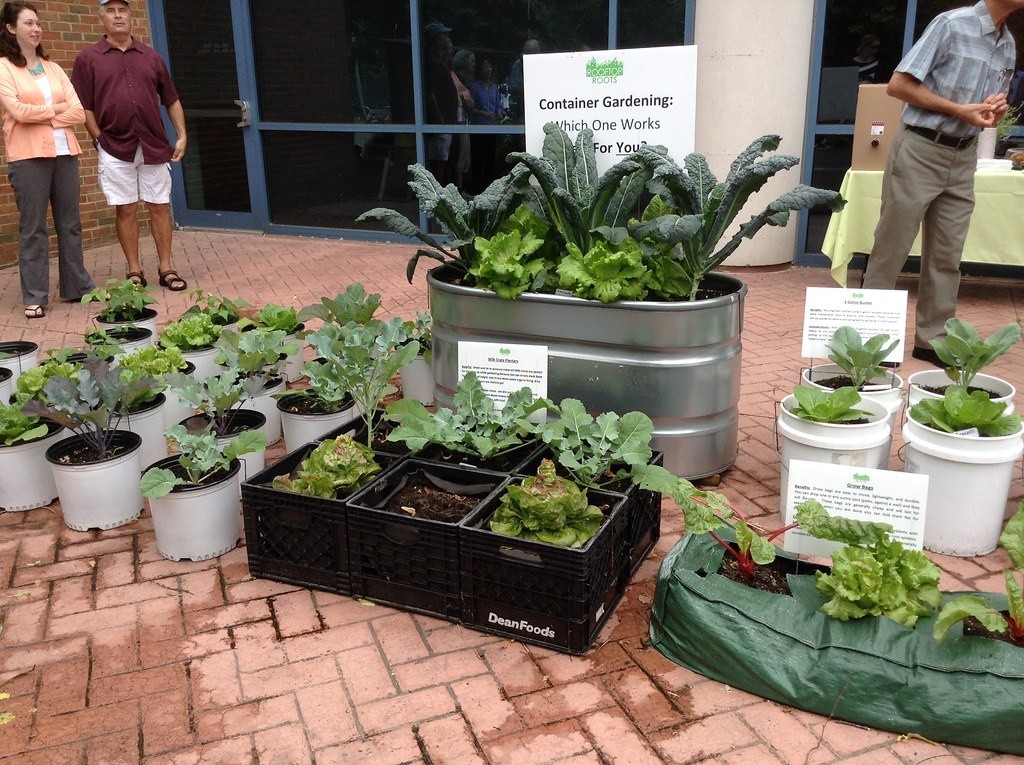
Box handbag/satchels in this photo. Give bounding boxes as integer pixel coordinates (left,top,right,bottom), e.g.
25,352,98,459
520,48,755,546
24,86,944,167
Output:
456,115,471,175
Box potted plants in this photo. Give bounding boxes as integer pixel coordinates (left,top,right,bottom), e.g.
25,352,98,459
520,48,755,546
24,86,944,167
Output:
0,119,1023,754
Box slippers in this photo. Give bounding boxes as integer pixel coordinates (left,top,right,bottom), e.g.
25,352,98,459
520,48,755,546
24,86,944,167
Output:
70,289,111,302
25,304,45,318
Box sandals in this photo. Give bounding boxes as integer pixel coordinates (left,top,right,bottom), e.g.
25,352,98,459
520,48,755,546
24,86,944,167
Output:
125,270,147,289
158,267,187,291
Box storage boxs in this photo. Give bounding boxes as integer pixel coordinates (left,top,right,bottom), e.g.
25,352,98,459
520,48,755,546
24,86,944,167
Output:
853,80,974,171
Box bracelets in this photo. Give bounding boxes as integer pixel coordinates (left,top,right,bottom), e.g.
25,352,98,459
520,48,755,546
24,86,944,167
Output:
93,139,100,151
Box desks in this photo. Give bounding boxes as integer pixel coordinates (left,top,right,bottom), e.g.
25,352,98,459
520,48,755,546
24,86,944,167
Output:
818,159,1024,288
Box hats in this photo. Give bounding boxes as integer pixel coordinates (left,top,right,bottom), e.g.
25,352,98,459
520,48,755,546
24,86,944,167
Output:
423,21,453,45
99,0,130,5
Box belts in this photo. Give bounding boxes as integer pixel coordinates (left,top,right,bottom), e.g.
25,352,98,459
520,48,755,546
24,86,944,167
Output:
906,124,977,151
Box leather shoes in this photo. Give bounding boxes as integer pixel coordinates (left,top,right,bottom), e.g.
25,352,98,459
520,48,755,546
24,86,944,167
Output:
879,362,901,368
912,345,967,370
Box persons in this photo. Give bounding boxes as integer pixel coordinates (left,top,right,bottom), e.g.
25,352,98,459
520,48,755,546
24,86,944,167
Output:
0,0,111,319
70,0,186,292
860,0,1024,370
852,35,880,83
423,22,592,199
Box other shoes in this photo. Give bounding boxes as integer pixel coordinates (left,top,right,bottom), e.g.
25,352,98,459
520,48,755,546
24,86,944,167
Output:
461,190,476,201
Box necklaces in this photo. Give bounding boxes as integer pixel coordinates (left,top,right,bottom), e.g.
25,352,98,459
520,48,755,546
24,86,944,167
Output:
27,62,44,76
